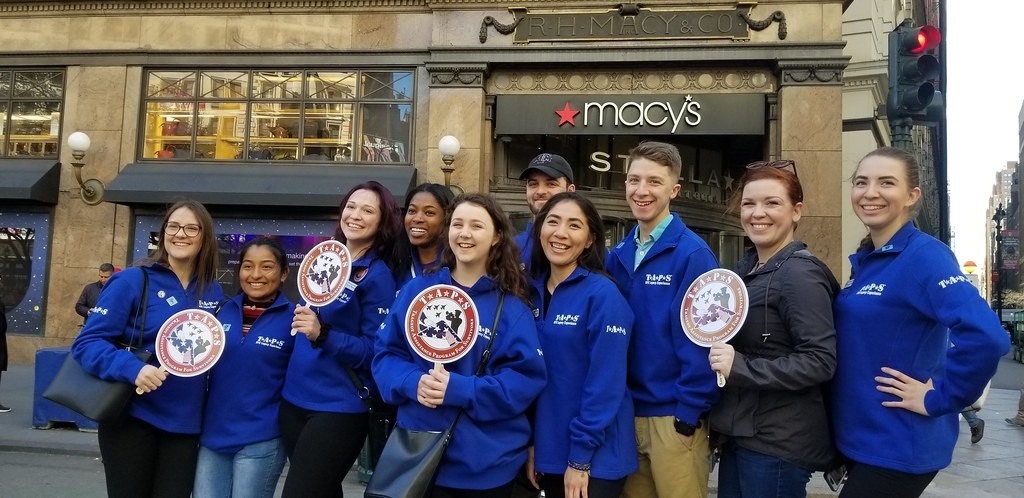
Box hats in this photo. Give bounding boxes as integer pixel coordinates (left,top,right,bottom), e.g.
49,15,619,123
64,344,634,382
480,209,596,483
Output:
519,154,573,187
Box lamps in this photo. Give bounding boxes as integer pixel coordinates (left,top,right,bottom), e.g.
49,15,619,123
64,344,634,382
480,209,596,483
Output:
68,131,105,205
438,135,467,201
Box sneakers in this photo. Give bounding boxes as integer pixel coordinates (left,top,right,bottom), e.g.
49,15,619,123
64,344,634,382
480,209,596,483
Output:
969,419,985,442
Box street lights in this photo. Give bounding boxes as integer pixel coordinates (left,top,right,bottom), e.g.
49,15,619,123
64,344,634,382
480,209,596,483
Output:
992,203,1005,325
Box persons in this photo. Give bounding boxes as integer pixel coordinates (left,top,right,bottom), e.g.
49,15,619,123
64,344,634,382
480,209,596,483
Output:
826,147,1011,498
75,263,114,324
705,159,840,498
604,142,719,498
1005,383,1024,427
963,410,984,443
192,237,296,498
72,200,222,498
279,153,639,498
0,275,11,412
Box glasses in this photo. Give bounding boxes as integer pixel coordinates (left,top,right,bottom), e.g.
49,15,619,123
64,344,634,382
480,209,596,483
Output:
163,220,202,238
744,159,798,182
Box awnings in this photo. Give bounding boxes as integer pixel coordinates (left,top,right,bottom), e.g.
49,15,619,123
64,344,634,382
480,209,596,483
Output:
103,163,417,208
0,159,60,205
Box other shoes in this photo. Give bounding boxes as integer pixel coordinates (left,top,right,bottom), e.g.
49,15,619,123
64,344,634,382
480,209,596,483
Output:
0,405,12,412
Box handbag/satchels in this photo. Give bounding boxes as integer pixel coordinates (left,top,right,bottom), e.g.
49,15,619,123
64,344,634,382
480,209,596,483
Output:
364,425,450,498
43,265,152,421
365,396,399,472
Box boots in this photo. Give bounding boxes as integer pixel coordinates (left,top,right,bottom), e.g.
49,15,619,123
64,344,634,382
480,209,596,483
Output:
1005,388,1024,426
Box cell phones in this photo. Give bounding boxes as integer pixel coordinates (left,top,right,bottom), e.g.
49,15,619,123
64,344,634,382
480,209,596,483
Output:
823,464,846,491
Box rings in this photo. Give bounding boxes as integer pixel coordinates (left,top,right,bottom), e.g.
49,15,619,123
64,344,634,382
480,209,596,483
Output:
716,356,718,362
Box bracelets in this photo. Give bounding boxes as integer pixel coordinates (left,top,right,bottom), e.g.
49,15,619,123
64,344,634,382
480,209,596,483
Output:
568,460,591,473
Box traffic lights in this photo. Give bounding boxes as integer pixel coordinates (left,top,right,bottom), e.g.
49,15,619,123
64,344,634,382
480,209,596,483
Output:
887,24,940,119
